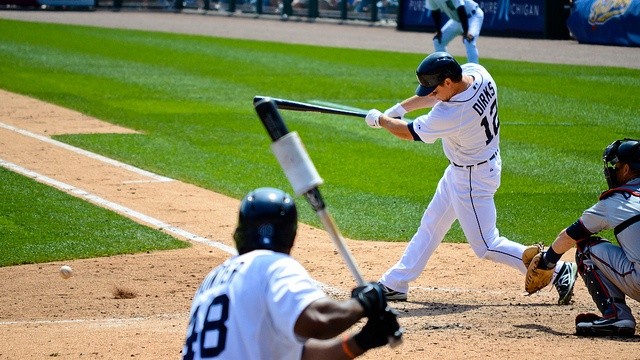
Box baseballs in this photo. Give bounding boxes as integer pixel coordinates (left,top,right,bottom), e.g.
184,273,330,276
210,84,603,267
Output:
59,266,73,280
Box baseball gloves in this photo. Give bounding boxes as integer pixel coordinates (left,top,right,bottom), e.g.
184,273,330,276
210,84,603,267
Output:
522,241,556,296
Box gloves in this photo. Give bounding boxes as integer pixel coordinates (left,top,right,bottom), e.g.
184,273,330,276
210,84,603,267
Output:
365,109,384,129
351,280,388,316
383,101,409,122
355,309,401,354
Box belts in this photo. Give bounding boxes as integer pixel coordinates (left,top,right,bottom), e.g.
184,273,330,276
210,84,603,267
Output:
448,149,499,169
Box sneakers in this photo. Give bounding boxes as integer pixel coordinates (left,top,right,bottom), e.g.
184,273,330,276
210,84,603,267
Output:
575,315,637,340
376,282,408,303
549,261,579,306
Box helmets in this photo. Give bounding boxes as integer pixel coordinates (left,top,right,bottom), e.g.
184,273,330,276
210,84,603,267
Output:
232,186,299,255
602,137,640,189
414,51,463,97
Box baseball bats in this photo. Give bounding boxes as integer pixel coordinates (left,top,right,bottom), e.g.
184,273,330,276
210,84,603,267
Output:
254,95,402,119
254,97,404,349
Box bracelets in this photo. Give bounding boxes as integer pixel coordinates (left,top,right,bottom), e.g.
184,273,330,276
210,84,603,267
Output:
342,336,355,359
546,245,565,262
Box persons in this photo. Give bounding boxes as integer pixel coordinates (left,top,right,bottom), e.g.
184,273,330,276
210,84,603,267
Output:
163,186,399,360
522,139,639,338
364,51,580,307
421,1,488,62
